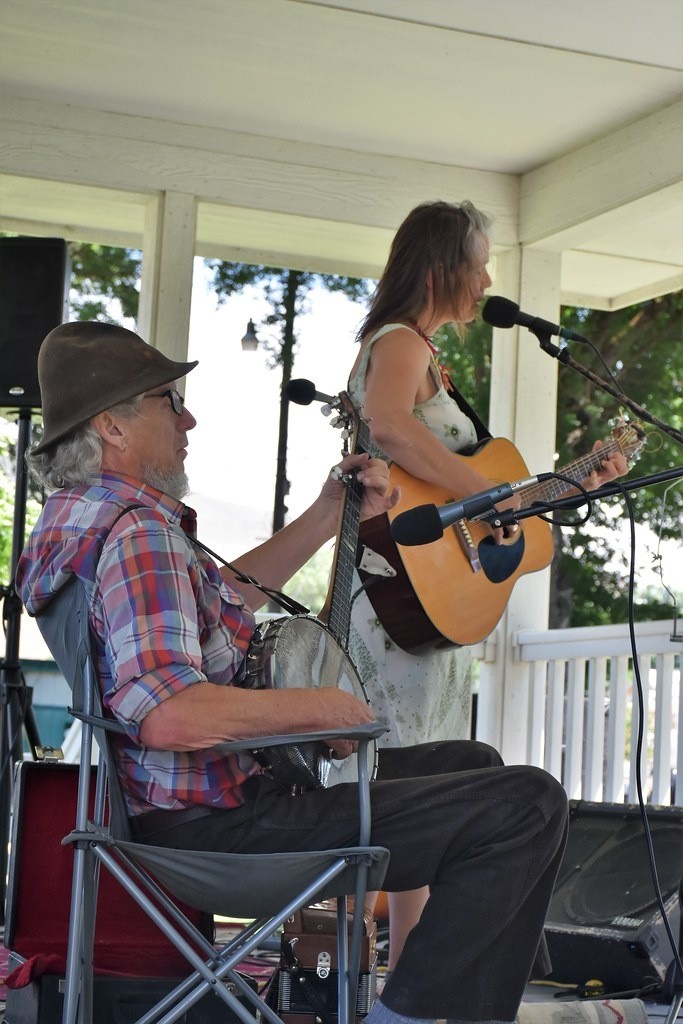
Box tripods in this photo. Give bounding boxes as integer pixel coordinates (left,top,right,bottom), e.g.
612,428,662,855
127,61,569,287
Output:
0,408,43,929
580,869,683,1024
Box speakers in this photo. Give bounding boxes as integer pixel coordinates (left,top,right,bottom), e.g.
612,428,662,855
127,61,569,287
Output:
0,236,70,421
529,799,683,988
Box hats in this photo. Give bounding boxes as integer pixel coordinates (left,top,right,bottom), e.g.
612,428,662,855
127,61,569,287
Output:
29,321,200,456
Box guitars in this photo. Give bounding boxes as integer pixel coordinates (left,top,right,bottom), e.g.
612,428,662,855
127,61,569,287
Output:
234,388,383,793
351,401,650,659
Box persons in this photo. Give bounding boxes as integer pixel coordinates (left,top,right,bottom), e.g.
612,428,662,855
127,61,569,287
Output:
15,321,569,1024
327,197,632,971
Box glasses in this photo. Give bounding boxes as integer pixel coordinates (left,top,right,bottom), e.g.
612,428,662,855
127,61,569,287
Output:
145,388,186,416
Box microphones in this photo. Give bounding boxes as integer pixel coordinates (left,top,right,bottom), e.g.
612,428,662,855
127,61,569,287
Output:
390,472,554,547
286,379,332,406
482,296,589,344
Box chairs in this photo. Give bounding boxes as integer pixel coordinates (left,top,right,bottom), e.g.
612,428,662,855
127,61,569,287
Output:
32,576,390,1024
